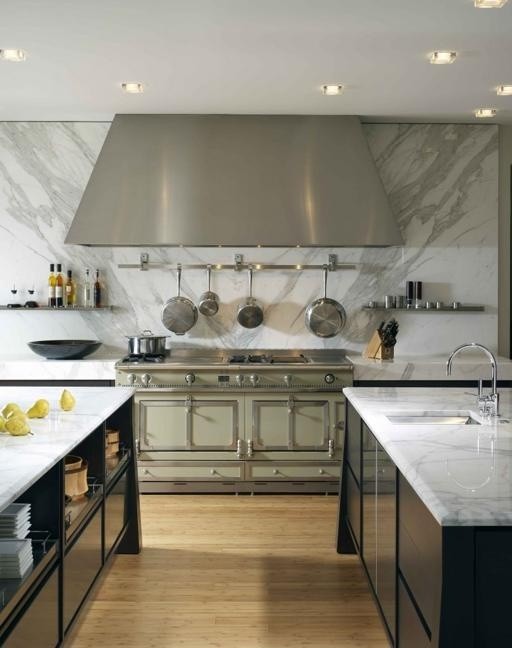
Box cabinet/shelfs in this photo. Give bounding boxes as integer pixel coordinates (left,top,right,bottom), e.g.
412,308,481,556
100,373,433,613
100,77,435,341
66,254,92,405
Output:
104,396,135,569
342,399,361,555
1,460,63,647
360,420,398,648
395,469,511,648
61,420,104,648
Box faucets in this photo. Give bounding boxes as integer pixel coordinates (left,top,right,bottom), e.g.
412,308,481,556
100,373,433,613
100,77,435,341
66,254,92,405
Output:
445,342,500,417
442,433,497,493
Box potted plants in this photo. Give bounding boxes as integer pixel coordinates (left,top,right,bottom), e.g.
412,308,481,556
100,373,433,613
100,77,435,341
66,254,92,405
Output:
377,317,399,360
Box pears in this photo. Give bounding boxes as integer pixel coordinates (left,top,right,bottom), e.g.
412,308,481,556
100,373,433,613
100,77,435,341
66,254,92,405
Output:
0,388,75,436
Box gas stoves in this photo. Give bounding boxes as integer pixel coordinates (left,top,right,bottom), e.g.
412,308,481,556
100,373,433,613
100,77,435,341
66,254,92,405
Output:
115,350,354,390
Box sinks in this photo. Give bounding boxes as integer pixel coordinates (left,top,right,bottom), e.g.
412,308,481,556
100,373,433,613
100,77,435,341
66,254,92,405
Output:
383,409,490,425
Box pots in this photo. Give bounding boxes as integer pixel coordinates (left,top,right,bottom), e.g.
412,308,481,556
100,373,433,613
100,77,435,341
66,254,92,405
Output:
161,264,199,335
238,268,265,328
124,330,170,356
196,265,220,316
304,265,347,339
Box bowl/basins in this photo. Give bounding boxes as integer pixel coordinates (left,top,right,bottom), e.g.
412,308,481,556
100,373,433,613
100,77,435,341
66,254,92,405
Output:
63,456,90,498
29,339,103,360
106,428,120,456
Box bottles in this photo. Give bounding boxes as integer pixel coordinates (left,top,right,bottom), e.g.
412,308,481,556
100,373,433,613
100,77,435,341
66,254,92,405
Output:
404,280,423,309
47,262,103,309
8,289,40,308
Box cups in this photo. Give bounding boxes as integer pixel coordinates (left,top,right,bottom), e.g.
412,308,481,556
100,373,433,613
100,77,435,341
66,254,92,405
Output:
425,300,443,310
384,294,404,309
367,301,375,308
452,302,460,309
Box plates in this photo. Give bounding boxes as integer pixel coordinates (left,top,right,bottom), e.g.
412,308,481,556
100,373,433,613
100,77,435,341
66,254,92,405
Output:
0,501,34,580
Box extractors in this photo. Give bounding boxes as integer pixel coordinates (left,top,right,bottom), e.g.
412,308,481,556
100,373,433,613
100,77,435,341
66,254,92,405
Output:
65,114,406,249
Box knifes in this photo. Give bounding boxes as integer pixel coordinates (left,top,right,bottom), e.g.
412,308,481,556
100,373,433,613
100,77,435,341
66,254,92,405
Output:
379,318,399,348
